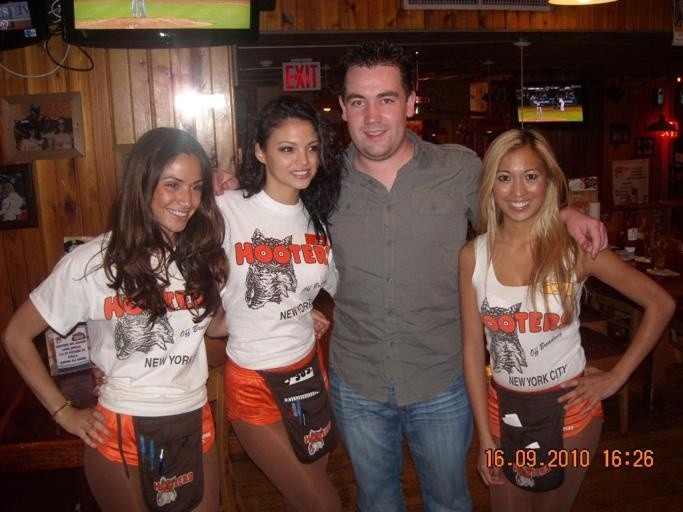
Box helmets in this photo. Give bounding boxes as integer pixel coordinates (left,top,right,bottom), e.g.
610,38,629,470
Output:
646,269,680,277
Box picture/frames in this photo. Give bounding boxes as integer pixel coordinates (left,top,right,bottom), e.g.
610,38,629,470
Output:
0,371,96,472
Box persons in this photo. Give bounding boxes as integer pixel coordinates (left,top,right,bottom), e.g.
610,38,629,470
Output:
132,0,145,19
21,124,43,151
0,183,24,221
2,127,230,512
303,37,609,512
14,103,41,151
38,115,54,150
54,117,73,151
457,128,677,512
211,95,344,511
528,89,576,120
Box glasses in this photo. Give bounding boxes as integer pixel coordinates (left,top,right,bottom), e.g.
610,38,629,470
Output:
646,105,679,131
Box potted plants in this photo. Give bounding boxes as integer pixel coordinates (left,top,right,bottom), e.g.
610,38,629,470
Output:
0,163,44,230
45,324,93,377
634,136,656,157
0,91,86,164
607,122,632,144
113,144,137,193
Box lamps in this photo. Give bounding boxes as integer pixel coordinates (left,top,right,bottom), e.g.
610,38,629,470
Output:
579,326,630,435
205,365,242,512
650,231,683,269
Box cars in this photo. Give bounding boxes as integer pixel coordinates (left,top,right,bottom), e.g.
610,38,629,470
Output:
653,240,667,271
589,202,601,221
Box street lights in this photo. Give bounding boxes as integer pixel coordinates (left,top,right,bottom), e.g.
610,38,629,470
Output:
264,353,336,464
495,390,564,493
132,407,203,512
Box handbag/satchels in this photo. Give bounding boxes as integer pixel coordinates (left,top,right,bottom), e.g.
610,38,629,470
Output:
52,398,73,421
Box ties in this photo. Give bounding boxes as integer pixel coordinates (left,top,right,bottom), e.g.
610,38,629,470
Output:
0,0,51,51
61,0,259,49
511,82,589,128
